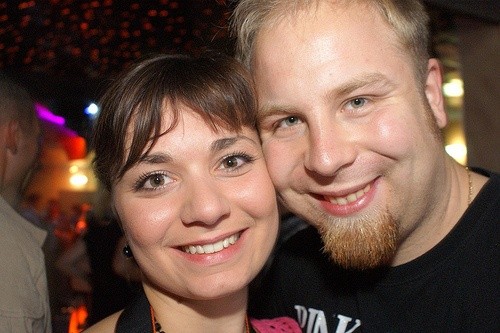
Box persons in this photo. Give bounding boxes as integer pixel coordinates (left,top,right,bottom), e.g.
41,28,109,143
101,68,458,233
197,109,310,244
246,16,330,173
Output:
0,80,51,333
82,55,302,333
229,0,500,333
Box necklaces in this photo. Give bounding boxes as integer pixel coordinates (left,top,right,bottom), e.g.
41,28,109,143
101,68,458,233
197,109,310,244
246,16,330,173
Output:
467,167,473,206
150,306,249,333
